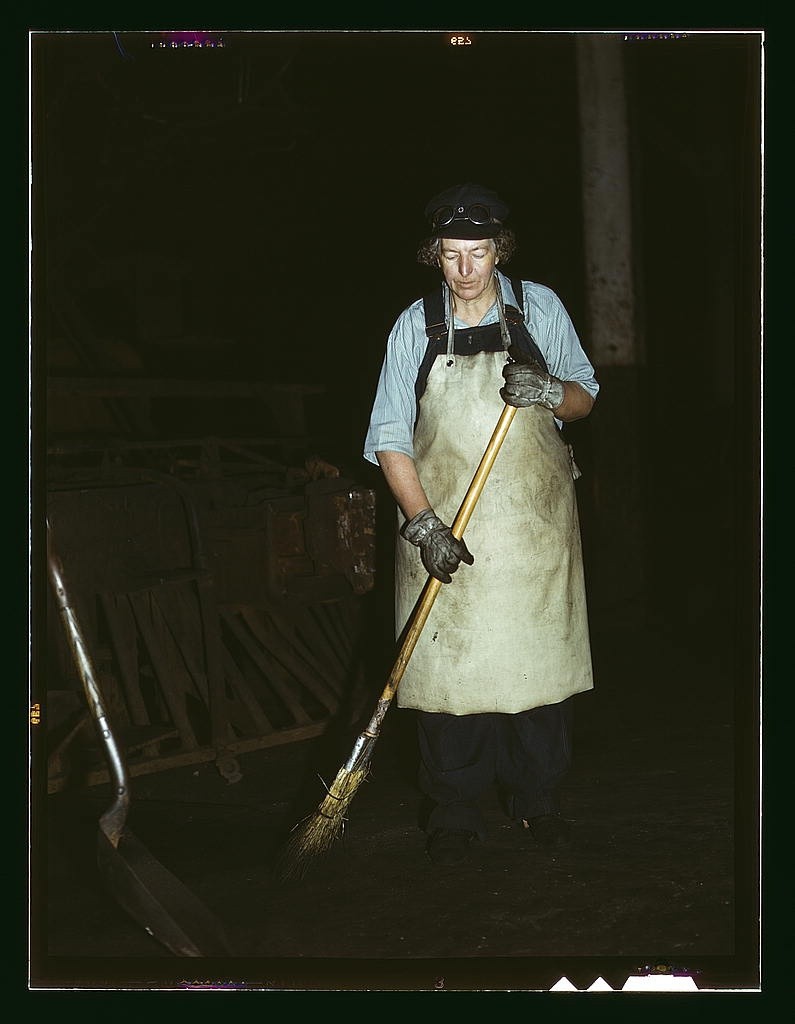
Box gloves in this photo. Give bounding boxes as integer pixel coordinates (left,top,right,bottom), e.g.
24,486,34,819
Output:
404,510,475,585
500,359,564,409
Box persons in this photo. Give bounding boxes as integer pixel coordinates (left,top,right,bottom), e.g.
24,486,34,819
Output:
364,183,599,866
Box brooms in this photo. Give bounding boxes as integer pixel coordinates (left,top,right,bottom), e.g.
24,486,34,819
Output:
269,404,519,889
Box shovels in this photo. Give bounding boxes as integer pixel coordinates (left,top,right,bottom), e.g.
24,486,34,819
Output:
45,554,228,958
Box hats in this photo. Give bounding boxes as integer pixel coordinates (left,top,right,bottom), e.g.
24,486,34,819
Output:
427,188,504,241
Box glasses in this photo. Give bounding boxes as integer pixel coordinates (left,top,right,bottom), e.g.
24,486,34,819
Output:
431,206,490,226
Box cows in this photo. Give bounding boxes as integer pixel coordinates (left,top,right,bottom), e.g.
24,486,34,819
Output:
306,459,380,594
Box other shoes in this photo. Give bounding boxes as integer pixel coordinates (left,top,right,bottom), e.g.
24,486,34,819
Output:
425,825,472,866
528,813,563,848
